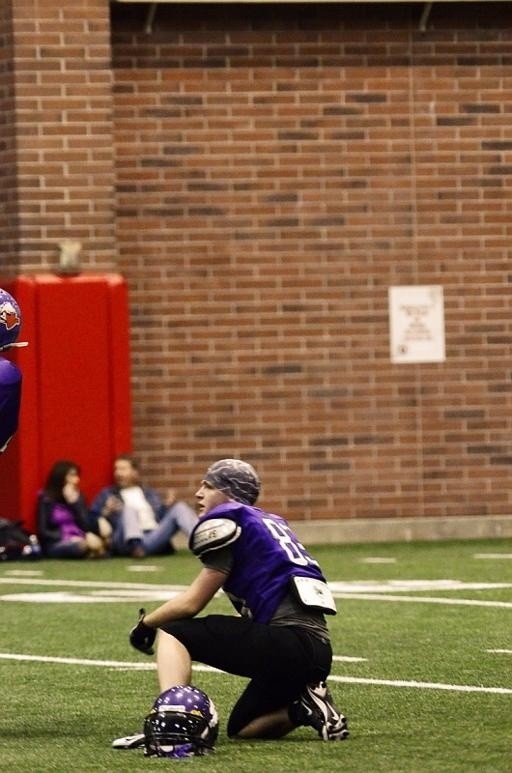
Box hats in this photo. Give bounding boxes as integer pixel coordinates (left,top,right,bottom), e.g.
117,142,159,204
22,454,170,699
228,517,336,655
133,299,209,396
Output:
205,459,260,505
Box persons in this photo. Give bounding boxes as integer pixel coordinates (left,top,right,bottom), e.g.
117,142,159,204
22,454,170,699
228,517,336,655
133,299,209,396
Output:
0,289,21,454
129,458,348,741
36,461,113,560
91,454,199,559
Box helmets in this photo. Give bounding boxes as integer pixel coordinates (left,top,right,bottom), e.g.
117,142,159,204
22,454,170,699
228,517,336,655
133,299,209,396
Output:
0,289,21,352
143,685,219,758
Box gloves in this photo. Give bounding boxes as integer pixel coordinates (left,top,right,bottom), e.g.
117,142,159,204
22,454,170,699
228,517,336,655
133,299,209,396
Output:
130,608,155,655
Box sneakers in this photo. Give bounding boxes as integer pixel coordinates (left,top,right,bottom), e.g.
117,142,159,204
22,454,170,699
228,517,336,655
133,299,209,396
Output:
111,733,146,749
298,681,349,740
